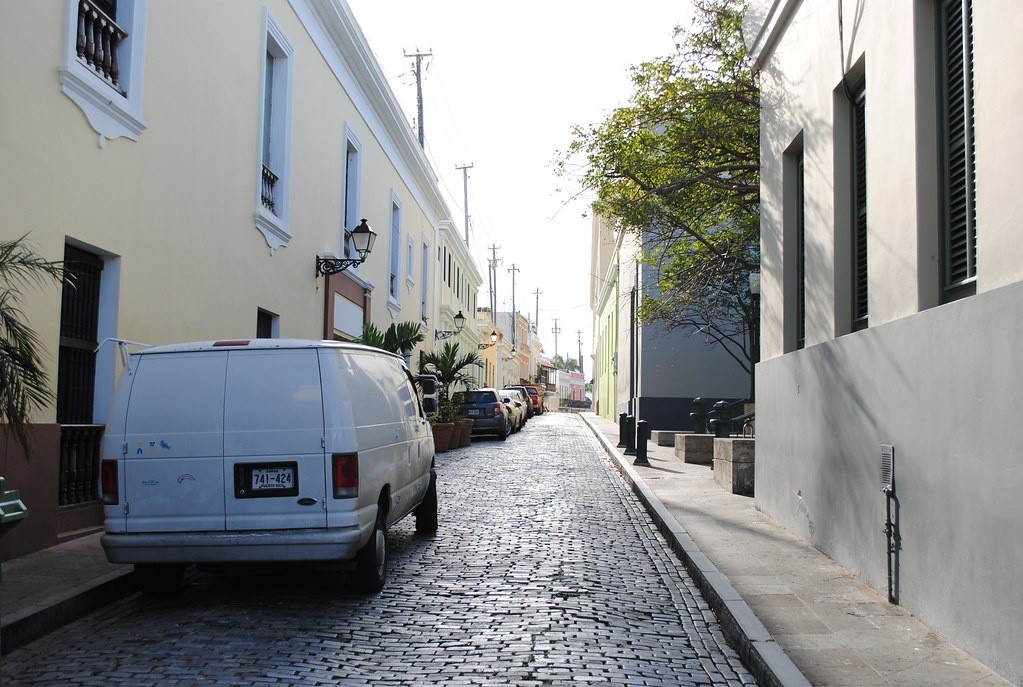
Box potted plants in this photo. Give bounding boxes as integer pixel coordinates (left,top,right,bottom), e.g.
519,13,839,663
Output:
422,342,486,452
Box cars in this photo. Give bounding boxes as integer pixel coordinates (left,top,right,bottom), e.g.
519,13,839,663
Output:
494,383,543,435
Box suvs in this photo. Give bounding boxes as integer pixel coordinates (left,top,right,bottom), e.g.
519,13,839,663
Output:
445,388,510,440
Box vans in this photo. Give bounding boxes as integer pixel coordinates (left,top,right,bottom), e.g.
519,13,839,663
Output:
98,339,442,594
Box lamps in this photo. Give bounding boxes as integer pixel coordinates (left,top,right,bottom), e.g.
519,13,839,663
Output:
503,346,518,362
316,219,378,278
435,310,466,341
479,330,499,352
